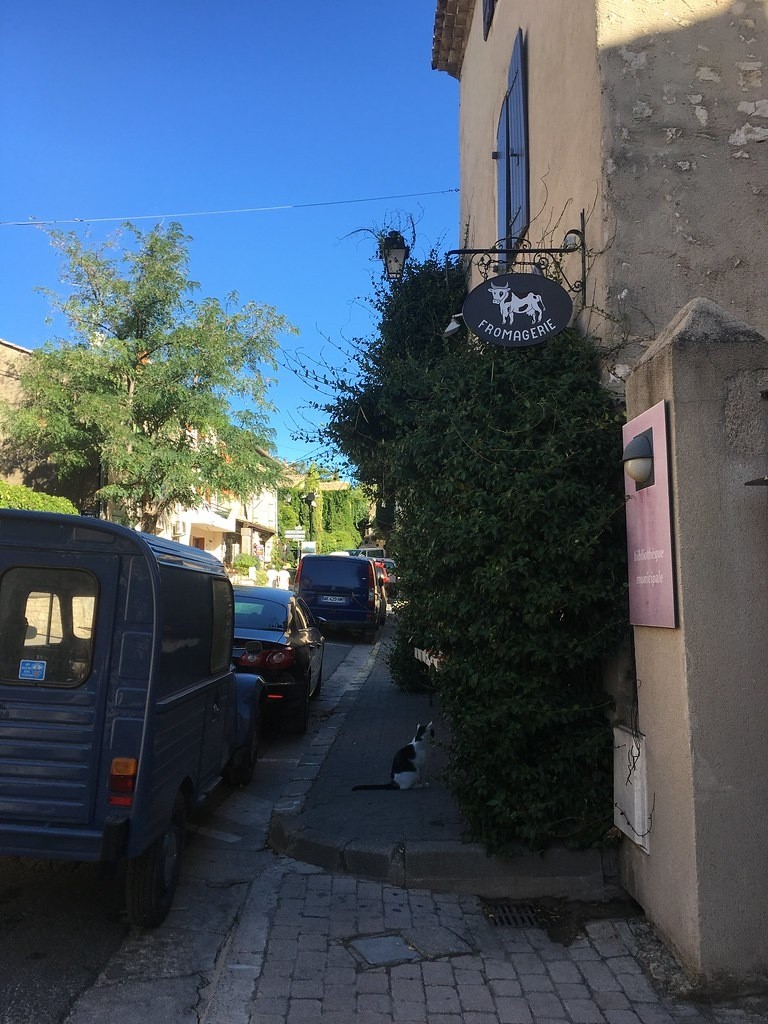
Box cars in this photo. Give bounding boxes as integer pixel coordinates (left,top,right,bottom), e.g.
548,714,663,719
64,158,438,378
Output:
341,548,400,626
220,584,327,735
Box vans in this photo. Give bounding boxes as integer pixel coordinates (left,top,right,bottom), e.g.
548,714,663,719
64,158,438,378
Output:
294,555,385,642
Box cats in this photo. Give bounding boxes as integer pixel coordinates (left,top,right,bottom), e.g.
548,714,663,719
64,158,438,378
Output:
351,720,435,792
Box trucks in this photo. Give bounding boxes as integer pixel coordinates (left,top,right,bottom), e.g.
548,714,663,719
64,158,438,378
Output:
0,505,267,931
